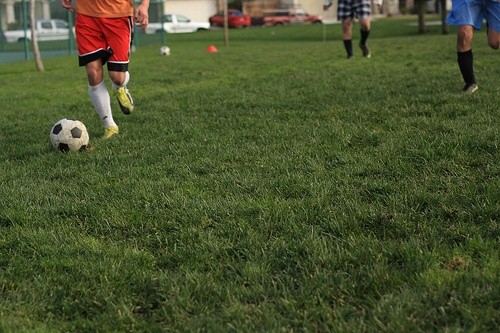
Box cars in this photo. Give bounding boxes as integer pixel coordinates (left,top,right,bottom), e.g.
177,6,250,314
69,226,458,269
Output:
208,9,251,29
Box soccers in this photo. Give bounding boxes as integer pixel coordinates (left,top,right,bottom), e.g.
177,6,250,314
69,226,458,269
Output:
159,46,171,56
49,118,90,155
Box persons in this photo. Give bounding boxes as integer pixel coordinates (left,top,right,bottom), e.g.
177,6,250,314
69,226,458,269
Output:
324,0,372,58
61,0,150,140
445,0,500,93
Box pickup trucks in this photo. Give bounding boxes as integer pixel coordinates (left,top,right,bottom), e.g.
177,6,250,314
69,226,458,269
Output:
143,14,211,34
3,19,77,43
263,9,323,26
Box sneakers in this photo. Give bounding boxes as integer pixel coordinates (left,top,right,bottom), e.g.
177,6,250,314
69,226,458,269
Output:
112,83,133,115
101,125,119,140
461,83,478,94
360,41,371,58
